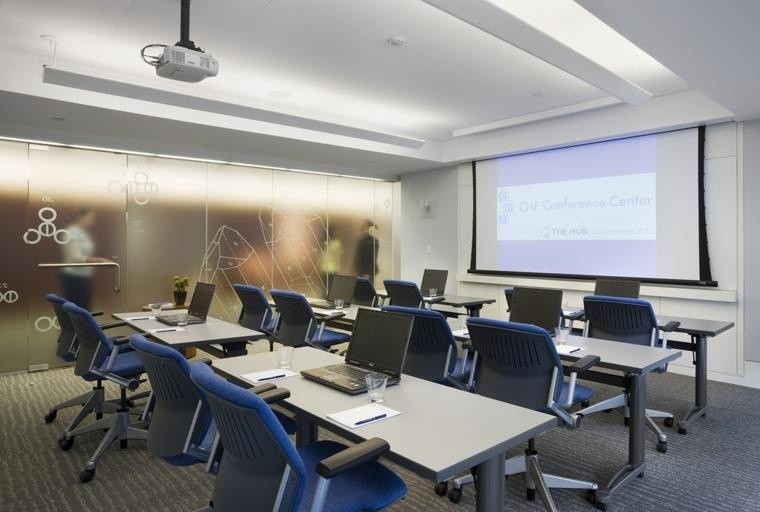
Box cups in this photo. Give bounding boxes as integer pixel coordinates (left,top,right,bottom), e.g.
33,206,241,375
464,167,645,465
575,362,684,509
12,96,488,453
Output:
334,298,345,312
174,312,190,332
149,303,164,316
457,314,471,331
364,372,389,404
275,345,295,374
554,326,570,345
428,287,439,298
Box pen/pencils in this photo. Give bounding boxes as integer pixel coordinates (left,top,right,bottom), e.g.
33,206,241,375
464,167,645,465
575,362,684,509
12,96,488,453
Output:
128,316,150,321
257,374,286,382
330,311,343,314
156,328,177,334
569,347,581,353
355,413,386,427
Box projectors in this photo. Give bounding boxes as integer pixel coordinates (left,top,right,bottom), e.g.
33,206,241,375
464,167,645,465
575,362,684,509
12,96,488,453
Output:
141,40,219,83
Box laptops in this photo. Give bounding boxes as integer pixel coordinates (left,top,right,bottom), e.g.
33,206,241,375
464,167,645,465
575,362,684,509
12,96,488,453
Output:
156,281,216,326
508,286,563,339
300,306,415,396
594,279,640,299
420,268,448,303
310,275,357,310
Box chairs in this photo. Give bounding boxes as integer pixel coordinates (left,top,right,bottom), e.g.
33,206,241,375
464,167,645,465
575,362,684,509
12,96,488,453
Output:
381,305,473,496
583,295,673,451
268,289,350,354
504,287,566,333
383,279,429,312
58,301,150,483
190,361,406,511
126,332,296,479
232,283,275,352
349,278,378,308
464,314,597,511
43,291,145,437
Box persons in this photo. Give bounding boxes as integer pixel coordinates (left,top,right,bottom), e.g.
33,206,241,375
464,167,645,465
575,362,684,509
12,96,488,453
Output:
56,206,114,360
317,223,343,299
352,218,379,288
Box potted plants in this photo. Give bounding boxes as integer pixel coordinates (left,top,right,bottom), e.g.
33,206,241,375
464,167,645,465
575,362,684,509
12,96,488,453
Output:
171,273,191,306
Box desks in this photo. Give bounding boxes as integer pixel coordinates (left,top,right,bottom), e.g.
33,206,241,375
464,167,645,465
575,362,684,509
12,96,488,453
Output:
450,319,681,510
113,308,267,360
376,290,496,326
562,306,735,435
203,344,558,512
